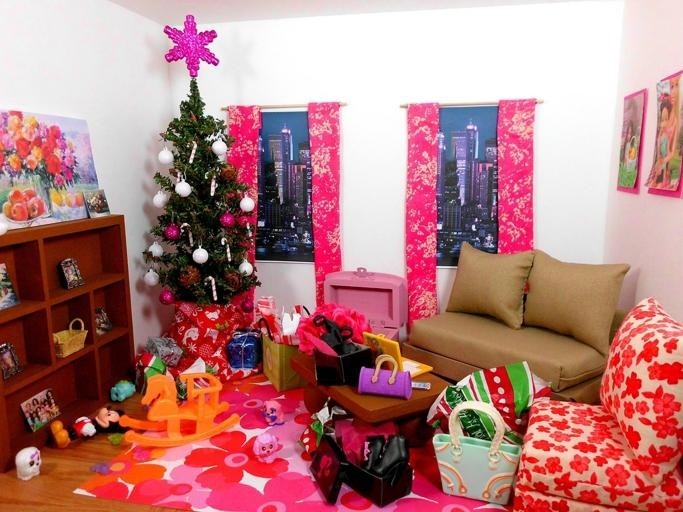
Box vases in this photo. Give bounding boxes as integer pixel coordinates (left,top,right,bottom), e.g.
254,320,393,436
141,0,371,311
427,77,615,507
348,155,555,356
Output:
8,171,50,220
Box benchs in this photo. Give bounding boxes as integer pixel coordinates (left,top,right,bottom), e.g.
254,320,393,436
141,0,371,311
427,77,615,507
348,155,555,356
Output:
399,303,627,405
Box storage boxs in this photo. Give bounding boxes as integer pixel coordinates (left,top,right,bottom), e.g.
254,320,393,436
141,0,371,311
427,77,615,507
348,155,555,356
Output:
261,332,305,392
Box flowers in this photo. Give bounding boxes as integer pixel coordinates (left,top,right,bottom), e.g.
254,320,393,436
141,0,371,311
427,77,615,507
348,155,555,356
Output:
0,110,78,193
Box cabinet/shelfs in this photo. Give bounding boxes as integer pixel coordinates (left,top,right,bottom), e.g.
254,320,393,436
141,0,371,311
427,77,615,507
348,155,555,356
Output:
0,211,138,473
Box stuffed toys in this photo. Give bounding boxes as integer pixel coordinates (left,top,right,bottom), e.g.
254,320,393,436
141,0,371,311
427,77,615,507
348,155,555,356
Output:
13,444,42,481
109,379,136,402
48,403,130,448
252,433,279,463
262,400,284,427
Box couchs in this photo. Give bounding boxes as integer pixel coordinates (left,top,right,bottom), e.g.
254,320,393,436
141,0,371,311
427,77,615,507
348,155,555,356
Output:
509,399,683,511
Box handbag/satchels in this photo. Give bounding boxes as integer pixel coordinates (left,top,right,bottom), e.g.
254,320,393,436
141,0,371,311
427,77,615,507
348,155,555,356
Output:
433,401,522,505
258,306,312,392
358,354,412,400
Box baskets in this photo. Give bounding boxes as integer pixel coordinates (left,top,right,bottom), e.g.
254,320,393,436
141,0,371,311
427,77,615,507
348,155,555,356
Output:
52,318,88,358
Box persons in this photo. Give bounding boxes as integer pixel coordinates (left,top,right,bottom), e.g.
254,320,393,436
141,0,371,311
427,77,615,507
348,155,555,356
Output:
19,387,61,432
651,99,676,187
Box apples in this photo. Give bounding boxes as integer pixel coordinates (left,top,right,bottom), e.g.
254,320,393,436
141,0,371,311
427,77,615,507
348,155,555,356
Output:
3,189,44,221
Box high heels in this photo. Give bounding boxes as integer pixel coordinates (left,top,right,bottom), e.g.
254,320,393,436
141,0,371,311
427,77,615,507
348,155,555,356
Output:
362,435,409,488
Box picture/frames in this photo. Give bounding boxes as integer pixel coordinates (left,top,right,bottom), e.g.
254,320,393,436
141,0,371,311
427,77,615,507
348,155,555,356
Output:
0,342,24,381
59,256,84,291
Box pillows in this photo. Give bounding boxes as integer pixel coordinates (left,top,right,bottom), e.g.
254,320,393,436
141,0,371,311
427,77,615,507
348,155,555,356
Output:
597,295,683,486
523,246,631,356
445,239,533,330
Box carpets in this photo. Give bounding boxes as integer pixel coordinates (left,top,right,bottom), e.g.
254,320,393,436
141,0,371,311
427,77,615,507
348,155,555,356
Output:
68,363,521,512
